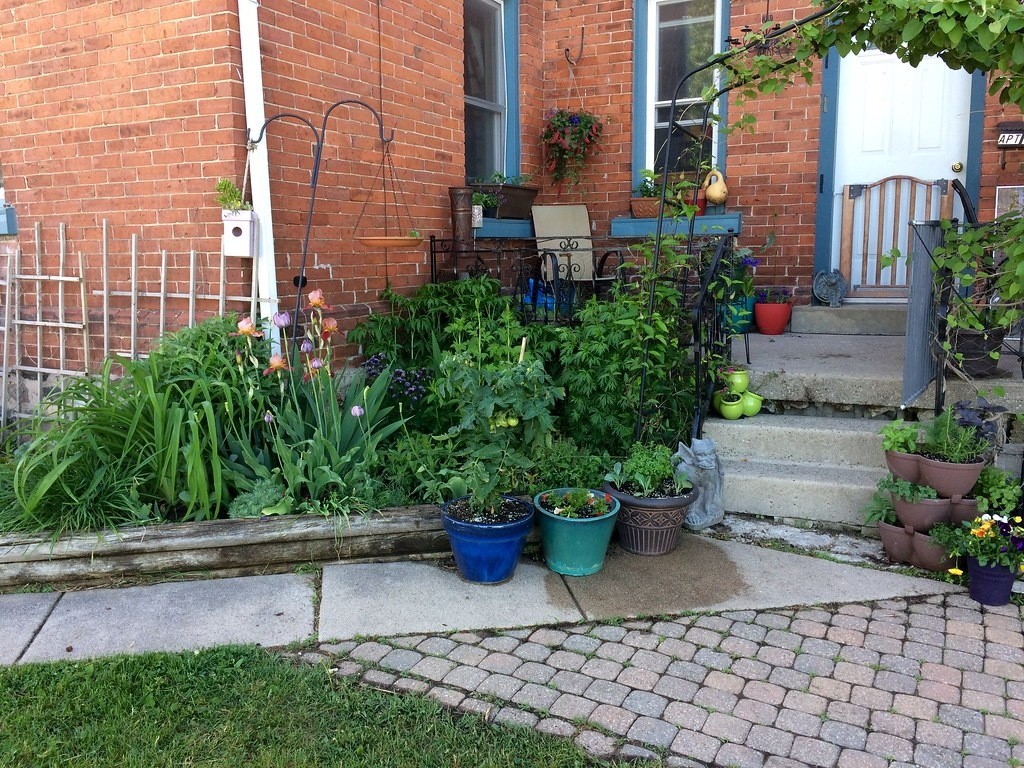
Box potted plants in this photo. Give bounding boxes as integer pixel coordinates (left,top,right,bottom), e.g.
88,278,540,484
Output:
629,168,669,219
532,437,622,576
472,192,498,227
355,230,424,248
467,173,540,220
409,351,568,586
708,364,764,421
862,402,991,571
602,439,699,558
215,178,257,257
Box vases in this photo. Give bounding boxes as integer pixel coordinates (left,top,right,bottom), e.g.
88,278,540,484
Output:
965,553,1019,606
678,188,708,217
946,326,1007,375
560,126,580,148
716,293,761,334
754,303,791,335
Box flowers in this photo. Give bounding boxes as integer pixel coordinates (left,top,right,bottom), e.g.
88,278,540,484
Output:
718,255,761,298
542,108,604,187
948,513,1024,575
757,286,793,304
